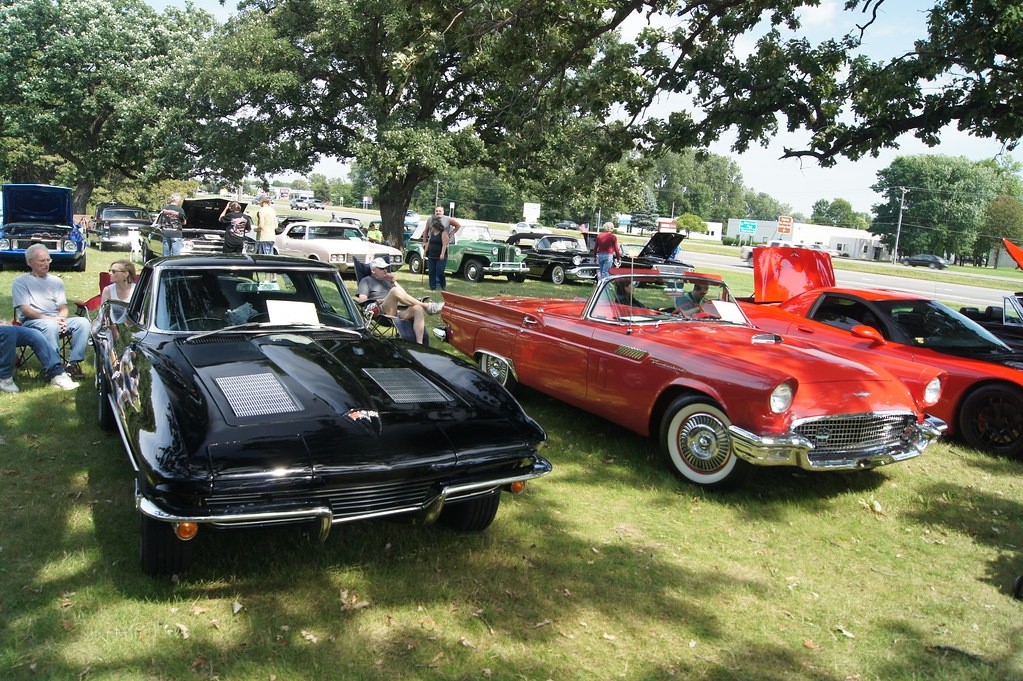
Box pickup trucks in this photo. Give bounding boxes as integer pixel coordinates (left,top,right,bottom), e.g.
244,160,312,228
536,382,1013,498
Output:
739,239,796,269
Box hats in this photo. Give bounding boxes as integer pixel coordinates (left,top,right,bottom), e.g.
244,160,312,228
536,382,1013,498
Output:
371,259,391,272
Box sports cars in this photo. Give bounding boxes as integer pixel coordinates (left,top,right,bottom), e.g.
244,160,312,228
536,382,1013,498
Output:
438,265,952,488
948,237,1022,365
732,246,1022,461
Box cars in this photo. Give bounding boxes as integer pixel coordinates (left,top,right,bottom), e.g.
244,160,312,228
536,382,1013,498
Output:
509,222,553,236
580,231,696,290
309,200,325,210
89,250,555,575
246,208,422,274
901,254,951,271
139,194,277,281
290,198,311,211
403,216,532,283
554,220,580,230
85,204,163,255
492,232,603,286
0,183,88,273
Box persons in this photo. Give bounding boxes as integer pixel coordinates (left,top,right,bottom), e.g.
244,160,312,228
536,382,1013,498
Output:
101,260,137,310
594,221,621,281
677,281,711,317
104,324,141,420
423,206,461,291
160,192,186,257
615,279,665,311
355,257,445,344
329,212,341,222
218,201,251,254
0,244,90,395
361,222,394,247
79,215,95,232
251,194,278,255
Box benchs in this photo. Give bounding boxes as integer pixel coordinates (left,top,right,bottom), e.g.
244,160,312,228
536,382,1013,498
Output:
546,304,620,319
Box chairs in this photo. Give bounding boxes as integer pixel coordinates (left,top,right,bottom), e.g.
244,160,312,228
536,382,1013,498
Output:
960,307,1004,323
849,300,889,338
11,271,139,380
352,256,429,346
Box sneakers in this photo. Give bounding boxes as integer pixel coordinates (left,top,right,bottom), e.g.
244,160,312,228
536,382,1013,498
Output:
0,376,18,393
50,372,80,391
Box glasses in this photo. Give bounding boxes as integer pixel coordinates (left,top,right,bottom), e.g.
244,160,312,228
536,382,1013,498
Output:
30,258,53,264
376,266,389,270
109,269,128,275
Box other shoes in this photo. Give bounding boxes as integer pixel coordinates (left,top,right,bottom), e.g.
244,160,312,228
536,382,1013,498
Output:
424,302,445,316
65,366,86,378
